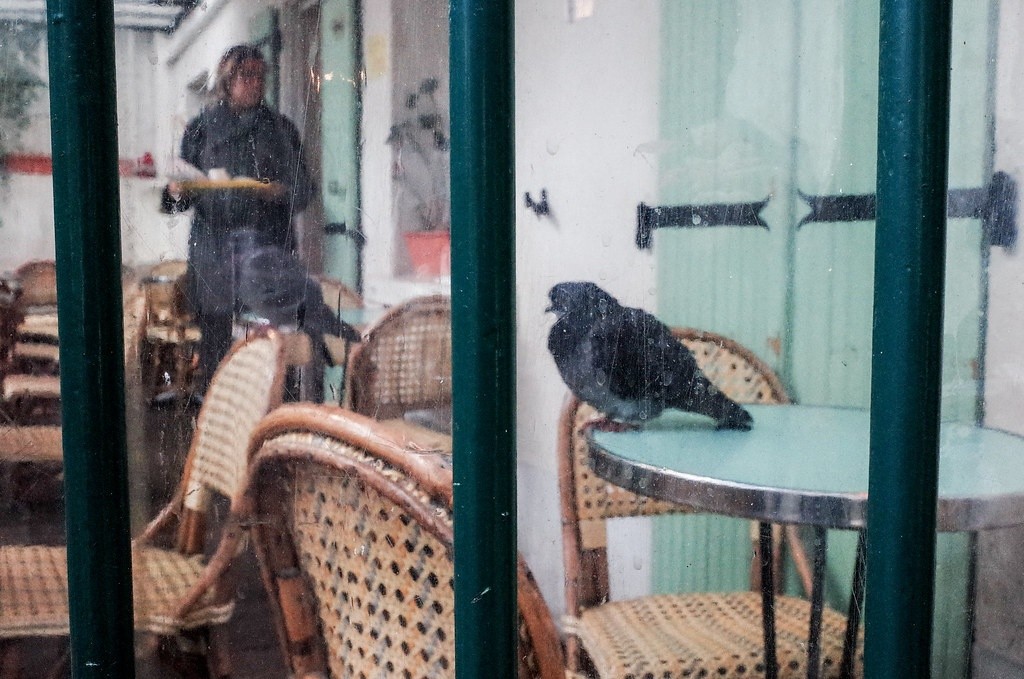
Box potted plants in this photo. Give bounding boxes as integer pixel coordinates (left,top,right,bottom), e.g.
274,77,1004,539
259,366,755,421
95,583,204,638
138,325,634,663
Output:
384,78,451,278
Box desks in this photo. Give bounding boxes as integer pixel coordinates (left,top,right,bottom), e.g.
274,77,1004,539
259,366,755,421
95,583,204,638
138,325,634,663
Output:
585,401,1024,678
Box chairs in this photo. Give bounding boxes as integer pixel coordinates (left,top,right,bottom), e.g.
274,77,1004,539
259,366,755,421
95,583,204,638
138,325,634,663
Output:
246,402,558,679
287,279,450,410
0,260,200,516
0,327,287,678
559,327,864,678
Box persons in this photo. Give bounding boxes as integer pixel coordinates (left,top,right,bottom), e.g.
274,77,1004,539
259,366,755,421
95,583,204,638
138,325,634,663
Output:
159,44,315,393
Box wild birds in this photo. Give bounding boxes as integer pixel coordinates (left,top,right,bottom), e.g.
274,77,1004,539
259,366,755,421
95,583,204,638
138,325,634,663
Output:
544,281,754,434
223,226,360,368
